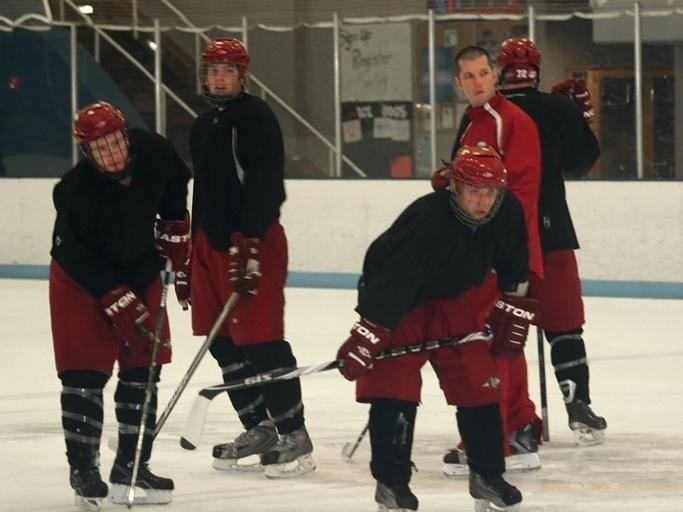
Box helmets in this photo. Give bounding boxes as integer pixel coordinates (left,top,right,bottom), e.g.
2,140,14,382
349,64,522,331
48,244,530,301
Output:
447,144,509,229
496,37,542,86
199,38,251,109
71,100,137,183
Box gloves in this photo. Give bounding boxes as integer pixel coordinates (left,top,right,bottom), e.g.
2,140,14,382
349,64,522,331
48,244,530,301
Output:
337,317,392,382
97,210,262,356
553,79,596,124
482,293,542,359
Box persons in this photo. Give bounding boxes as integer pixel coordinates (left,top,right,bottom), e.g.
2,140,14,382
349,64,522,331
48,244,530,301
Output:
336,140,530,508
450,36,606,439
46,99,191,497
431,47,542,467
174,35,313,469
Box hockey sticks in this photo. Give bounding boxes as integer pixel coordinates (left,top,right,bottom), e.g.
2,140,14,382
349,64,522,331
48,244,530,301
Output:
342,418,368,462
179,331,492,450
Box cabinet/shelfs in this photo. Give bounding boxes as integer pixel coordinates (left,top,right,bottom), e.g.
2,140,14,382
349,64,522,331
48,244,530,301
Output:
565,67,674,180
